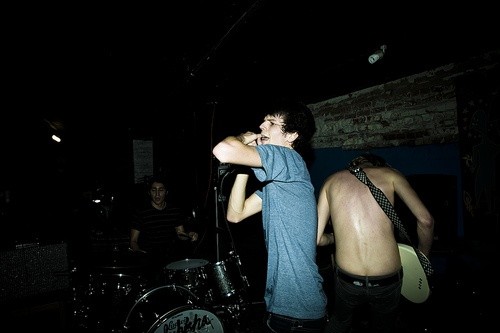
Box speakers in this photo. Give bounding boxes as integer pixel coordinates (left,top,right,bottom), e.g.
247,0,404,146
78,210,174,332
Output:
0,242,68,303
202,187,264,261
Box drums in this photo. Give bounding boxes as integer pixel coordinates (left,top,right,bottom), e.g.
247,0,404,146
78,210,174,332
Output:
124,285,224,333
208,262,243,297
163,259,209,286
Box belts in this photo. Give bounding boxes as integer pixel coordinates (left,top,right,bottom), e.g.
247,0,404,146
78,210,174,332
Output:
266,314,327,329
336,268,403,288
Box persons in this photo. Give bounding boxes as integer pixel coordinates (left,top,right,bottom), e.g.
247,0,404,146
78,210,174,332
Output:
212,97,328,333
130,176,199,267
316,152,436,333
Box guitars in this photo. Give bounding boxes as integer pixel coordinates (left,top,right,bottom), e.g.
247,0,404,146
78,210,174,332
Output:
396,242,430,303
